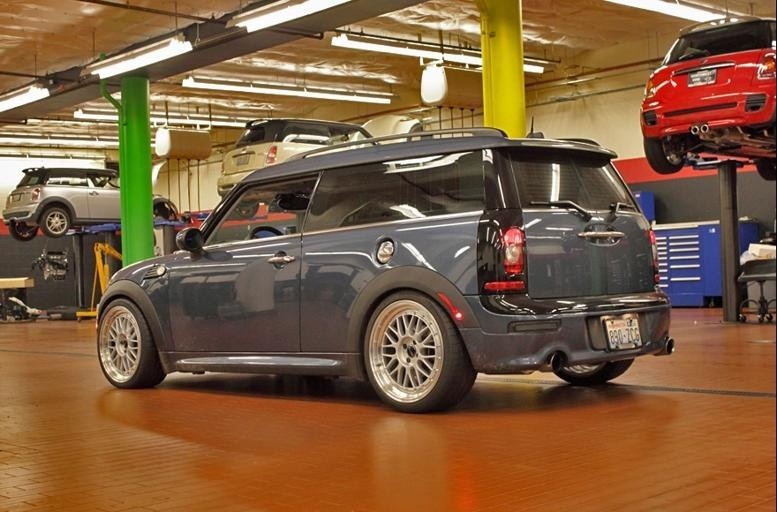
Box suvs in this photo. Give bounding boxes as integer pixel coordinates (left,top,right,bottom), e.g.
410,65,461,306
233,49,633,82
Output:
93,115,677,417
2,166,179,242
639,12,777,183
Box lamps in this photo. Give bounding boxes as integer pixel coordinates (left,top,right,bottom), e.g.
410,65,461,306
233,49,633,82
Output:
608,2,741,23
182,62,399,104
0,100,249,150
331,30,562,74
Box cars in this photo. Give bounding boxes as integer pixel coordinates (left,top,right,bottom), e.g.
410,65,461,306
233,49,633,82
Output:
217,116,379,196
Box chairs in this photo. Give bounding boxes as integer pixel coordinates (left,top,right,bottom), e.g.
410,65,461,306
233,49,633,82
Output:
737,238,776,323
